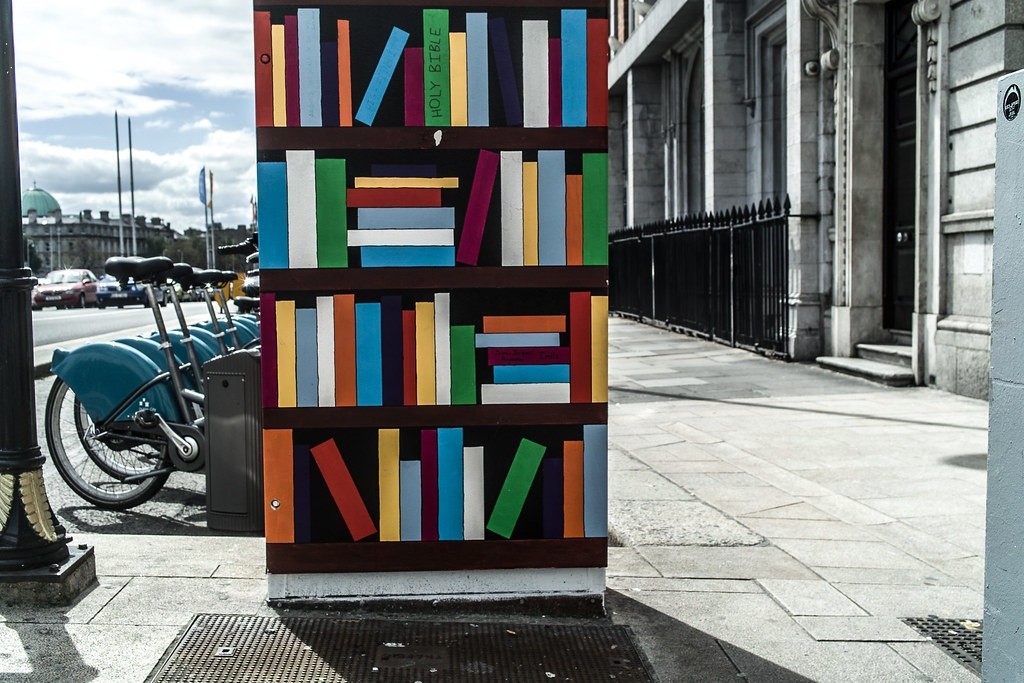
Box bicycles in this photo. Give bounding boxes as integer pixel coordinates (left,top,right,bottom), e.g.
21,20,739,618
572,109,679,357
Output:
39,234,258,509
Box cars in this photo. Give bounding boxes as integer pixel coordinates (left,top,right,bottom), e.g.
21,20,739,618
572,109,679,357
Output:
31,268,100,311
163,275,234,302
98,273,170,309
